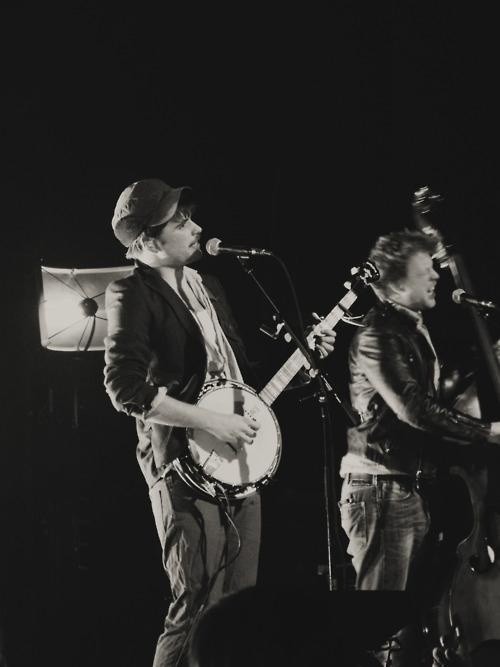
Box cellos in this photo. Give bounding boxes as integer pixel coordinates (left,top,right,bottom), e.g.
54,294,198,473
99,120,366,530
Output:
414,186,500,647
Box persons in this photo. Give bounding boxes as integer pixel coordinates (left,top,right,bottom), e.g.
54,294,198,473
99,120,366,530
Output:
337,226,499,590
431,561,499,667
103,176,336,667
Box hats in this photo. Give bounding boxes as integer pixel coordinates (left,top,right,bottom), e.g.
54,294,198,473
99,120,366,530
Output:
111,179,190,248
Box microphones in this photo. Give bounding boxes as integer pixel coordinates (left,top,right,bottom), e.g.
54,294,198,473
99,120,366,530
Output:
452,288,496,310
206,238,273,257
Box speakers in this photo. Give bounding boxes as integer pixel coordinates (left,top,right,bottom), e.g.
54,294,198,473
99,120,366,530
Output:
189,581,425,667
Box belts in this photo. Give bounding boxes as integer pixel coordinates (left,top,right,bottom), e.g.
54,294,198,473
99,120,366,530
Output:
350,474,417,484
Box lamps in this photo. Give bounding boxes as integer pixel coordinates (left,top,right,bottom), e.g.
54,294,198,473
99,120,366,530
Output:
33,253,147,632
